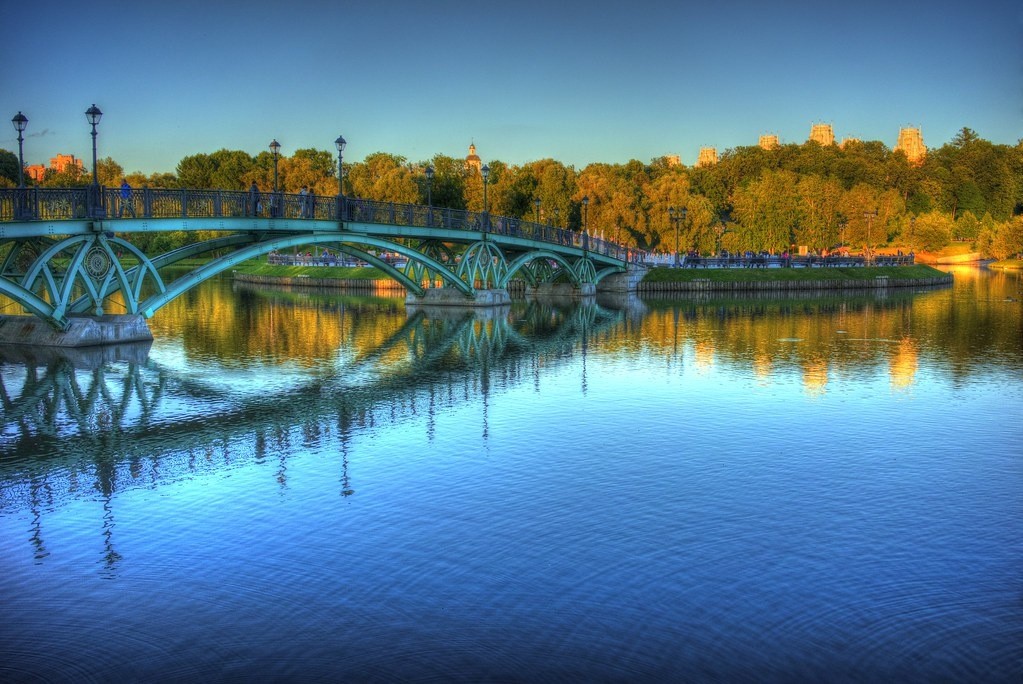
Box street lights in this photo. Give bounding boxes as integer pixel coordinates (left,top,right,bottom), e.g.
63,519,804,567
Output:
582,195,589,250
269,139,281,193
534,197,541,233
334,135,348,221
84,104,105,219
480,164,491,232
11,111,33,220
667,205,688,268
425,167,436,226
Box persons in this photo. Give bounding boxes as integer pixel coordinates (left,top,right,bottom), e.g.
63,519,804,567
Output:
779,250,788,267
556,226,569,243
843,250,851,257
832,249,840,257
115,179,135,218
858,249,863,257
319,249,329,266
897,249,903,256
270,252,275,260
295,251,302,266
721,248,767,267
381,251,390,258
510,221,530,237
330,252,335,259
249,181,260,216
347,194,362,220
299,185,316,218
687,247,700,268
909,249,914,255
303,252,312,266
474,219,502,234
806,251,810,259
